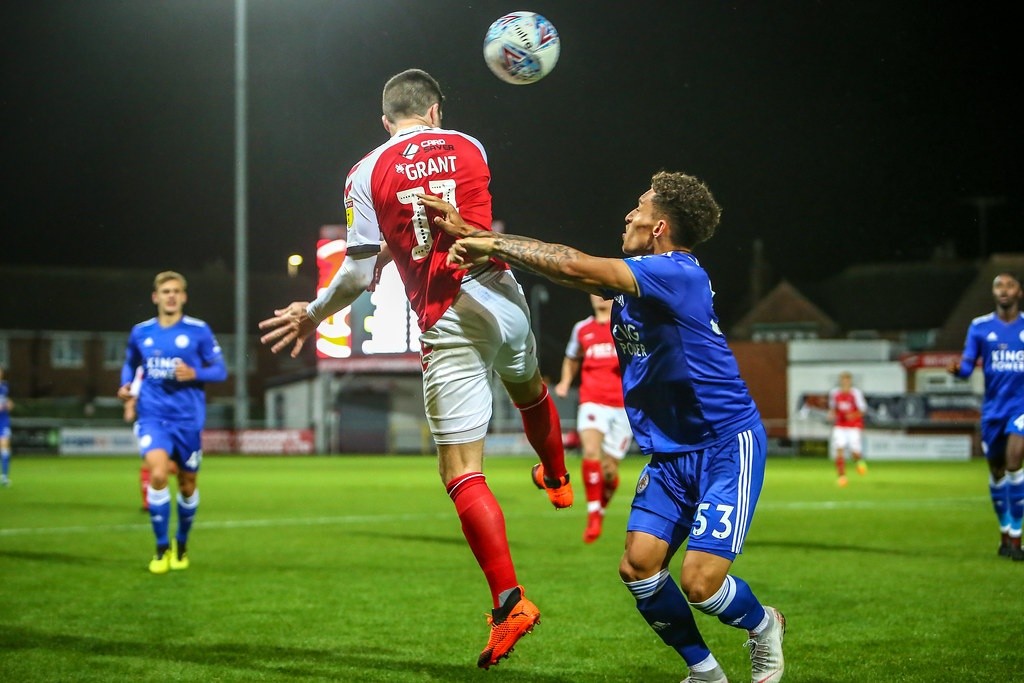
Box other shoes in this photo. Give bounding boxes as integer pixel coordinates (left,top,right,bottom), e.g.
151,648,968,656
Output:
856,460,867,474
839,476,846,486
602,478,619,509
999,545,1012,556
1012,547,1024,561
583,511,603,544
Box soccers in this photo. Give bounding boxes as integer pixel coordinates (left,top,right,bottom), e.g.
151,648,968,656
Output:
483,12,561,85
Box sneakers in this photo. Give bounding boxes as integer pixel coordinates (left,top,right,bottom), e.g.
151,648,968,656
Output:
148,547,170,572
680,665,728,683
531,463,573,510
478,583,541,670
171,539,189,569
743,605,784,683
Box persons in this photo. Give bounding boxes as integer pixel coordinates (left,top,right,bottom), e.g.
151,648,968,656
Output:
828,373,868,486
118,272,228,573
0,363,15,486
416,173,786,683
259,68,574,671
947,272,1024,561
554,289,634,544
